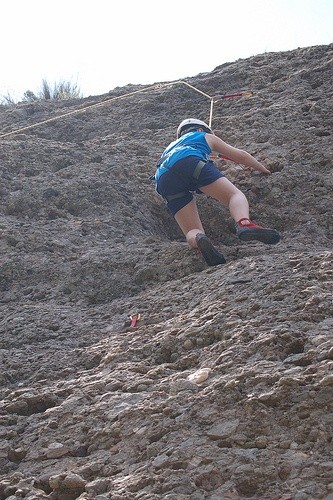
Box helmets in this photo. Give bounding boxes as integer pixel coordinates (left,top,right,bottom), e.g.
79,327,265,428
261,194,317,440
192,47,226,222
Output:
176,118,212,138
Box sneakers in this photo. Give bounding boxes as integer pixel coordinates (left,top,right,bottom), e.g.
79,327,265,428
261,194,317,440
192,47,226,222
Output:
234,217,281,246
195,233,228,266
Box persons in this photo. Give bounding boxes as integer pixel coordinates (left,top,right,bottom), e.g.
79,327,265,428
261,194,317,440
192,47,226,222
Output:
155,118,281,267
